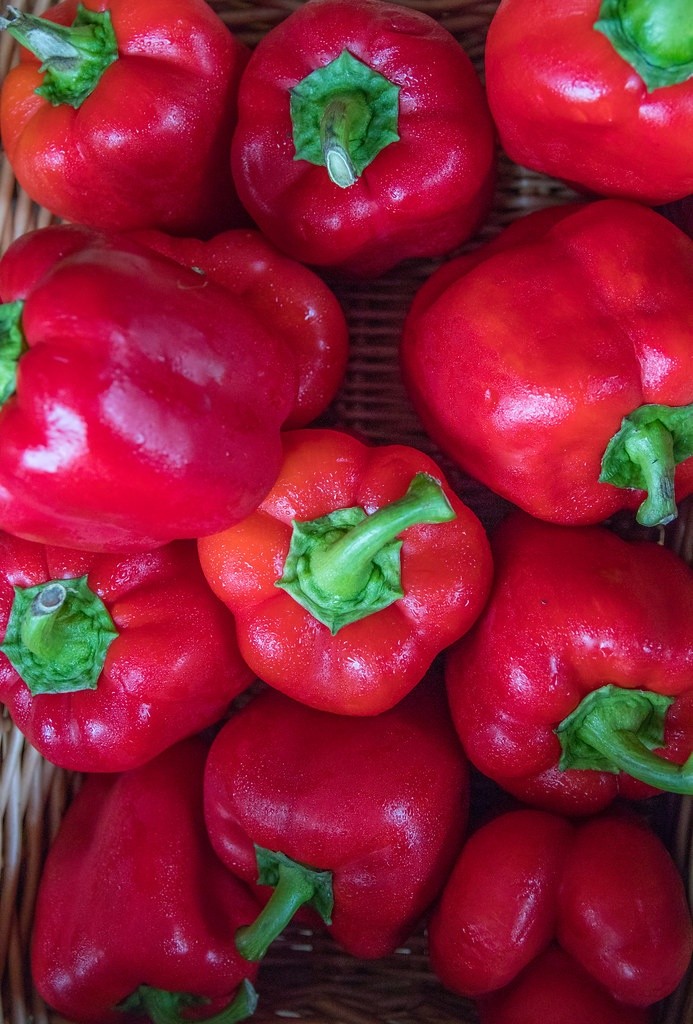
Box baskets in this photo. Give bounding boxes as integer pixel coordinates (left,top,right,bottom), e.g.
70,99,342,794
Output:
0,1,693,1024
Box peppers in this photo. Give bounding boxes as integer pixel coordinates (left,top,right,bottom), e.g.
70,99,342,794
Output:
0,0,693,1024
